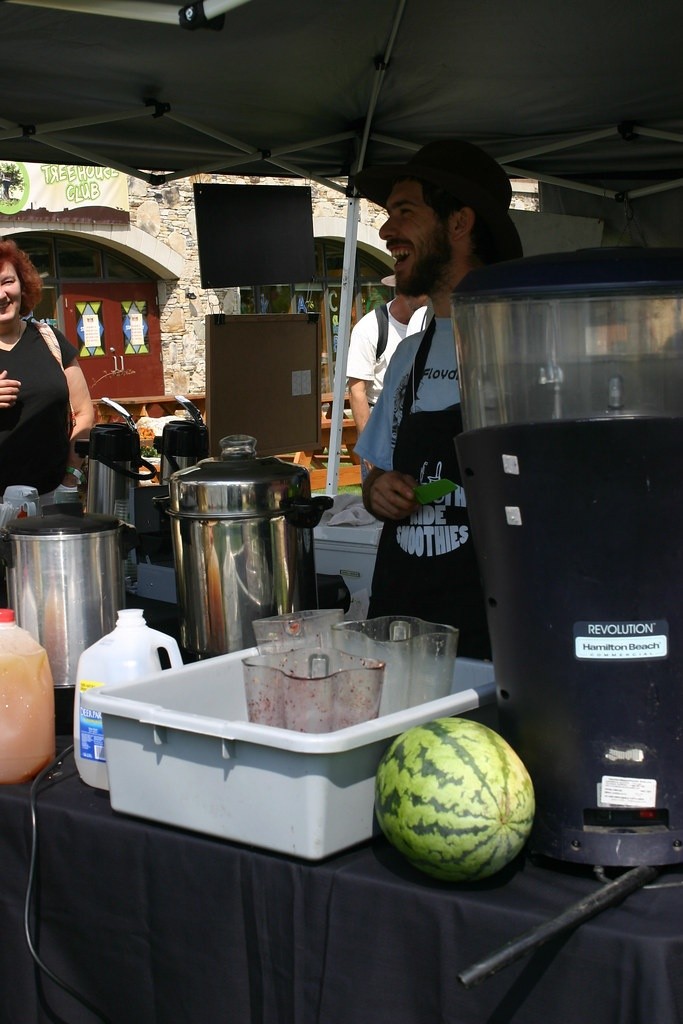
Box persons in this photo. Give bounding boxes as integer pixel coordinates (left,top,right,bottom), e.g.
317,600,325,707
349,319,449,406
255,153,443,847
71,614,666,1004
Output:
343,273,436,489
348,136,524,665
0,235,94,514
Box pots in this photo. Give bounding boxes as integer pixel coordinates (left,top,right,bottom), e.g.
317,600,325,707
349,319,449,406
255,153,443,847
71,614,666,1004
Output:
1,502,139,688
164,433,333,656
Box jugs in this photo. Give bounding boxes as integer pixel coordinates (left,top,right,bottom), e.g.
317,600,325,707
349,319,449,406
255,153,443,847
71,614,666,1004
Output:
153,394,209,494
74,398,157,532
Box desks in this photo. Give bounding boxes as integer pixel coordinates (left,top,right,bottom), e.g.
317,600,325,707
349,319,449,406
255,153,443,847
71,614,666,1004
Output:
91,393,206,423
0,728,683,1024
273,391,361,462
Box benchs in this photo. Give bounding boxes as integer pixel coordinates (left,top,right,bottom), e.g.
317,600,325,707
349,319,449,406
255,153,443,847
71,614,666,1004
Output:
293,416,363,488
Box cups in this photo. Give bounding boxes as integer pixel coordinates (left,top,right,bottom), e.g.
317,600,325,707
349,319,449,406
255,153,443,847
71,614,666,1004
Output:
331,615,459,716
252,607,344,652
242,644,385,732
3,486,42,517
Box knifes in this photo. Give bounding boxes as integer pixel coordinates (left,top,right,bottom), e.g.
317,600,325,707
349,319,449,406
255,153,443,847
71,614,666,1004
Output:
411,479,458,506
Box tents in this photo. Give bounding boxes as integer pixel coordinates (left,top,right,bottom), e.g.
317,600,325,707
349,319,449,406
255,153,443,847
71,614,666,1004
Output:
0,2,682,499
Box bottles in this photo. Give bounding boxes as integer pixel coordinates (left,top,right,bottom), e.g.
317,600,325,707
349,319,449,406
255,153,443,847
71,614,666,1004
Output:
72,608,183,791
0,608,56,783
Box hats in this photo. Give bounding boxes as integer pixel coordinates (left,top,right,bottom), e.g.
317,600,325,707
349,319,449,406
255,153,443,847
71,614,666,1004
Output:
401,139,523,265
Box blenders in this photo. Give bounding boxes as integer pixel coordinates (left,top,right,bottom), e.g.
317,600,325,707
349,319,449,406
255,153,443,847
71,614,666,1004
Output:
451,242,683,867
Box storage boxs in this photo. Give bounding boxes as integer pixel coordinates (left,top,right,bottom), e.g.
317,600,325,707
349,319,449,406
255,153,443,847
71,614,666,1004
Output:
75,639,506,862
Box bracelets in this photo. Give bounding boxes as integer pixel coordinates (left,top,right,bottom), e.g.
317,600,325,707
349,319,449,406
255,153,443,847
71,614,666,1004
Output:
67,465,88,487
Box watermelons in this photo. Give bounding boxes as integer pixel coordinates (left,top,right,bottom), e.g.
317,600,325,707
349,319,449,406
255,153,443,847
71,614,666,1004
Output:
375,715,535,882
